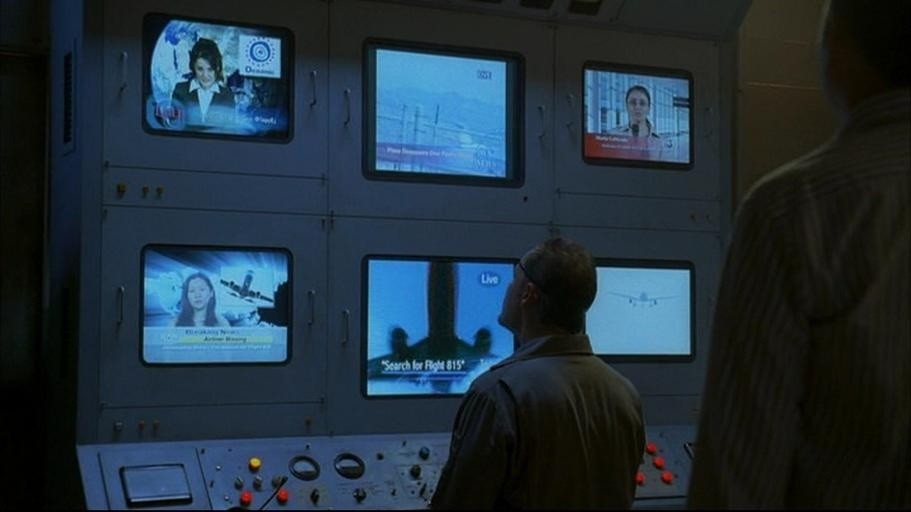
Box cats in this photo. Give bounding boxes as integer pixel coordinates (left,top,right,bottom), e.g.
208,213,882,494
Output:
608,290,679,308
220,269,274,303
366,262,505,394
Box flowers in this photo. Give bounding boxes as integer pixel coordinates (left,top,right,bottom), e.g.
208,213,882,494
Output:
632,124,639,136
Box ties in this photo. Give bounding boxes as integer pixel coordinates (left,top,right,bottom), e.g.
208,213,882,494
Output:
584,257,696,364
581,60,694,170
361,38,526,188
143,12,295,143
362,254,519,400
140,243,293,367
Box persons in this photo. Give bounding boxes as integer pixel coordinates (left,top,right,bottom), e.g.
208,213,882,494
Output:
170,37,236,132
428,235,645,512
169,273,232,328
681,1,911,512
603,85,663,161
